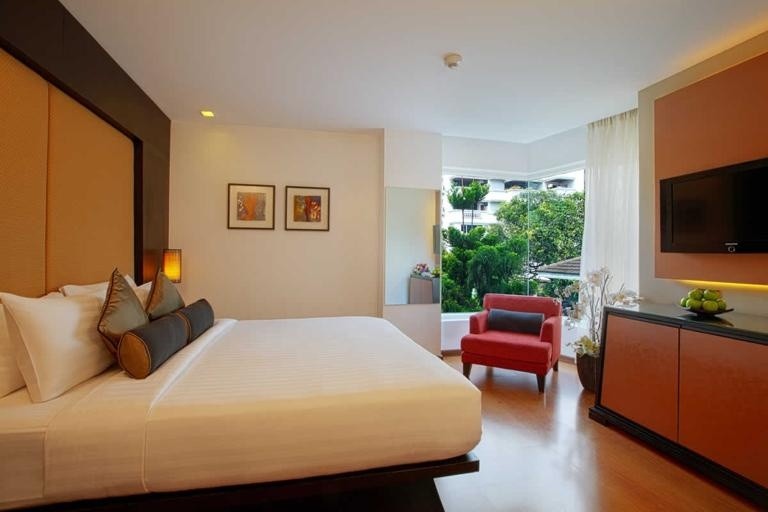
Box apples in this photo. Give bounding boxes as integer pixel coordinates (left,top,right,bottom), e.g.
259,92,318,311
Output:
688,287,703,301
680,297,689,306
716,299,725,308
715,290,722,299
686,298,701,308
703,289,717,299
703,300,718,310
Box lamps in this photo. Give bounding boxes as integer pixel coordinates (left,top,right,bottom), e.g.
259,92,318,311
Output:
164,249,181,284
443,52,464,70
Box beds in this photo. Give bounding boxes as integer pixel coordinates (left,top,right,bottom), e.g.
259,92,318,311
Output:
0,316,483,512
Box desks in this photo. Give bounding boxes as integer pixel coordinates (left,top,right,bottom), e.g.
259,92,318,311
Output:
409,275,440,304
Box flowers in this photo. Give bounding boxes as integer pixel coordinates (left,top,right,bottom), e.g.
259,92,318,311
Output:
412,264,430,276
561,266,644,359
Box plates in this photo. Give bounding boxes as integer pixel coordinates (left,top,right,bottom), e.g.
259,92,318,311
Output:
673,300,735,316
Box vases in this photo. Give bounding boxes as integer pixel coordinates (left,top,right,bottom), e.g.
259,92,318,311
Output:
576,351,603,393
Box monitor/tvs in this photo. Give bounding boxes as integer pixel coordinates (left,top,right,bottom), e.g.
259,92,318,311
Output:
658,157,768,254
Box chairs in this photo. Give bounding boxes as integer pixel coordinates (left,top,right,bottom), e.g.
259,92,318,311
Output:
460,294,563,393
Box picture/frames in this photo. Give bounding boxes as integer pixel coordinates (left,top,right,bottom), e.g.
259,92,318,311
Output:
285,186,330,231
228,183,275,229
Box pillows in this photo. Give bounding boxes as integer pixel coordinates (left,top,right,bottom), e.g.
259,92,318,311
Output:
132,280,154,312
0,291,119,404
488,308,545,335
117,313,189,379
0,305,27,398
56,274,137,298
96,268,151,363
145,265,185,322
174,298,215,343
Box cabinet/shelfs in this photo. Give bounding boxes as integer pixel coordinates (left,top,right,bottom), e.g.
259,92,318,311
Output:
588,302,768,512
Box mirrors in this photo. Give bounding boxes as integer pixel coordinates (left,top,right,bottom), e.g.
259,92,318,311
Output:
383,186,442,305
